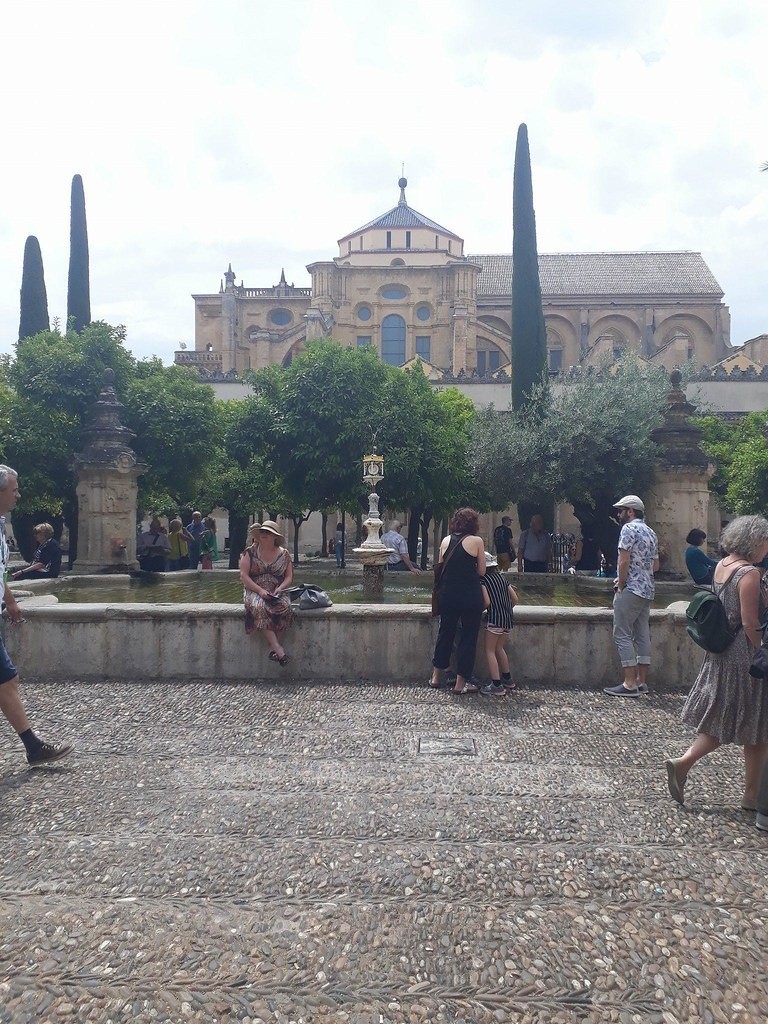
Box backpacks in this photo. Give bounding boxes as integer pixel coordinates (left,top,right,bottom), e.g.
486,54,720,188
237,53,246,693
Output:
685,564,752,654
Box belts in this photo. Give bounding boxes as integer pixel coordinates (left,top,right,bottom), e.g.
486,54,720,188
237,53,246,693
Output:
388,560,404,566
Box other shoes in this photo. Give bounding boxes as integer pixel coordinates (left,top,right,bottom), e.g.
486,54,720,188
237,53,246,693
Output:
480,681,506,696
279,655,292,668
488,675,515,688
268,651,279,661
756,812,768,832
666,758,687,803
603,684,640,697
741,796,768,815
615,681,649,694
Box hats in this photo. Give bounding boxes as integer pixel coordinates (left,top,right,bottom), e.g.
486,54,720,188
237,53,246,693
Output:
249,520,285,546
484,551,499,567
612,495,645,513
502,516,514,523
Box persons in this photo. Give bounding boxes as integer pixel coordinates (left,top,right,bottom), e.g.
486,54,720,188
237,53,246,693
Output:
480,551,518,695
565,524,601,576
429,508,486,693
665,515,768,832
685,528,718,583
494,516,516,571
381,520,424,575
518,515,555,573
239,520,292,665
563,544,577,573
136,511,219,571
603,492,660,697
330,523,348,567
0,463,76,765
11,523,62,581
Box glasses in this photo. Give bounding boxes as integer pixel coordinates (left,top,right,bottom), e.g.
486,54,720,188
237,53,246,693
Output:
258,529,274,535
614,508,627,514
398,525,403,528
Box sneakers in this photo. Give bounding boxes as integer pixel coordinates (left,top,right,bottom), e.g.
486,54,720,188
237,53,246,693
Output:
26,741,76,767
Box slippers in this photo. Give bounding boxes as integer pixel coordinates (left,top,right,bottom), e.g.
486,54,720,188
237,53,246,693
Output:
452,681,477,694
428,673,441,688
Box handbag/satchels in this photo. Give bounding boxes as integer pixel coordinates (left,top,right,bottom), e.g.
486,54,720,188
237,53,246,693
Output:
136,558,146,570
508,550,516,562
278,583,333,610
748,642,768,679
178,556,190,567
431,589,440,617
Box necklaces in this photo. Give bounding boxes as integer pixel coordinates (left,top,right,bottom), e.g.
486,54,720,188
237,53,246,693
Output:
722,558,748,567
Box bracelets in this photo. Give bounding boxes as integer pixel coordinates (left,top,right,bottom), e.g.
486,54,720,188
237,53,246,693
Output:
21,569,24,574
